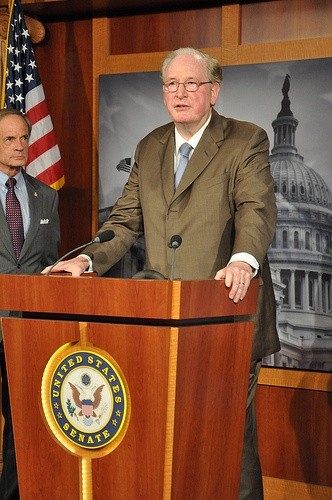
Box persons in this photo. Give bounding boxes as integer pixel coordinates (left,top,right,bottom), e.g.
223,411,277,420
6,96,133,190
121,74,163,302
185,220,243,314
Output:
41,48,282,500
0,108,61,500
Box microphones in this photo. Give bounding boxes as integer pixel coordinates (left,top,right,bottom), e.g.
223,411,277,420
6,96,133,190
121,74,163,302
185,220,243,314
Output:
169,234,182,280
46,230,116,275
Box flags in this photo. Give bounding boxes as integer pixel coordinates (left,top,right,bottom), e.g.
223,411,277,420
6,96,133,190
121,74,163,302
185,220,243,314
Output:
0,0,65,190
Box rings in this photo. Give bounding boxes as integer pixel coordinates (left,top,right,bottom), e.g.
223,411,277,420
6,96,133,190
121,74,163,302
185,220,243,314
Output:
239,282,245,285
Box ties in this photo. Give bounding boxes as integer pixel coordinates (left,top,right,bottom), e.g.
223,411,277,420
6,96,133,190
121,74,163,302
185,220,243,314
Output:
174,143,194,190
5,178,25,261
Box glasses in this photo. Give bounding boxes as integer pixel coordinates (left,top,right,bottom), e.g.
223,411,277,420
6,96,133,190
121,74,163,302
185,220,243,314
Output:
162,79,212,93
